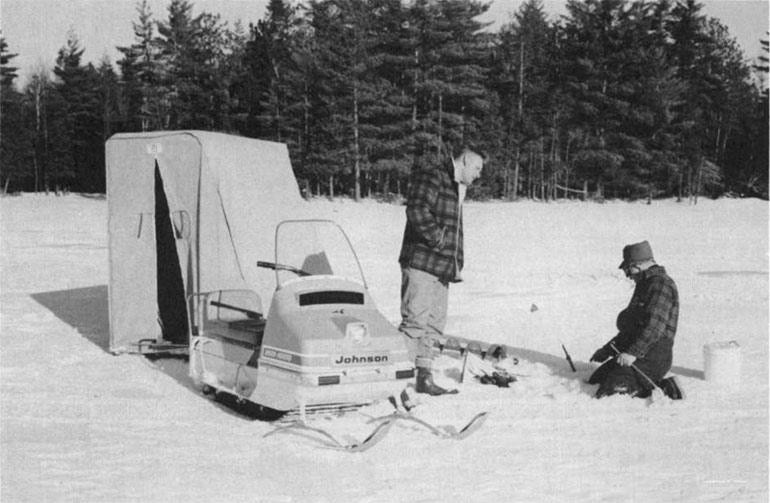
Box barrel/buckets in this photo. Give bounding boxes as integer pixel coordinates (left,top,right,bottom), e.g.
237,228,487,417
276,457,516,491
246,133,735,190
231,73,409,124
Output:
703,340,740,384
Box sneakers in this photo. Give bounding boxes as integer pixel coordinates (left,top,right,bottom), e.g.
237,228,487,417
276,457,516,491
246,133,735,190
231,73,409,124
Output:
664,374,686,400
416,377,459,396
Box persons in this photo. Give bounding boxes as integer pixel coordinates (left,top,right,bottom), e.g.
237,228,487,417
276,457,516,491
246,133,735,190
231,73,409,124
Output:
396,140,495,398
587,239,687,402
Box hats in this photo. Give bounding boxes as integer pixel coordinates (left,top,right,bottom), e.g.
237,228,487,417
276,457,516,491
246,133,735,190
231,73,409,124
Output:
617,240,654,269
